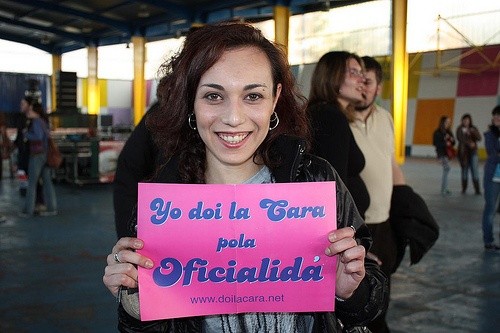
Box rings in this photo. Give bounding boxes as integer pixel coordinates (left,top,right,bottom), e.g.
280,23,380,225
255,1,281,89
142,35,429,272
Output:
114,253,122,263
351,226,355,232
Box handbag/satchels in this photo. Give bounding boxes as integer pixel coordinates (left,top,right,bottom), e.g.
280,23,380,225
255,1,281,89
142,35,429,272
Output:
29,122,45,154
47,139,61,168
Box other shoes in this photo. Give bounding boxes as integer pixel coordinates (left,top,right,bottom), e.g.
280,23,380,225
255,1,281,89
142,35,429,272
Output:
39,209,57,216
484,244,500,253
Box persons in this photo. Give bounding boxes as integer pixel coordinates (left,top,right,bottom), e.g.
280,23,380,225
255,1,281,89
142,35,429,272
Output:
457,114,482,195
103,23,367,333
482,105,500,253
346,56,406,251
0,97,57,223
306,51,383,267
433,116,456,195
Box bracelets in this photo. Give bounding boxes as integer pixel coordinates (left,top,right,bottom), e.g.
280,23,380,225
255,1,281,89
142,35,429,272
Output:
335,296,344,302
